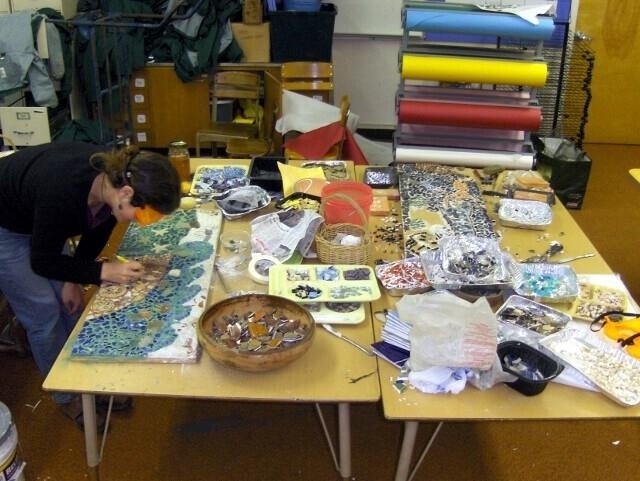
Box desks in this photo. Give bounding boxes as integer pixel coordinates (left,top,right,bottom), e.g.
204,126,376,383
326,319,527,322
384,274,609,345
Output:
629,168,640,183
42,159,381,481
355,164,640,481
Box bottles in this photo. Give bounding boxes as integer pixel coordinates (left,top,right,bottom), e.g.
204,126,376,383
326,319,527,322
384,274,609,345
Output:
1,52,19,92
169,142,192,181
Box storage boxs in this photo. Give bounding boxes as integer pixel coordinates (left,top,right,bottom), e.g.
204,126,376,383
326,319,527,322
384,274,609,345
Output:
229,23,271,64
267,4,338,64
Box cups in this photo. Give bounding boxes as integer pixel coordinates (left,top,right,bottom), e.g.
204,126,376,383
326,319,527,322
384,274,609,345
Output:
220,230,252,275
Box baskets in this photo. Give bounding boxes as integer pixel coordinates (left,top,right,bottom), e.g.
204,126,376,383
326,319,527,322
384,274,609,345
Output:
315,193,376,267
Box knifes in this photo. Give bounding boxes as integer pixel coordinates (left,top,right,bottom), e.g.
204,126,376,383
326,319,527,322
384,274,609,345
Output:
320,322,374,358
556,251,595,265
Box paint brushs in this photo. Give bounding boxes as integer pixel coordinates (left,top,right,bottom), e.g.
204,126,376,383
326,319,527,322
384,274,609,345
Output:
115,254,129,264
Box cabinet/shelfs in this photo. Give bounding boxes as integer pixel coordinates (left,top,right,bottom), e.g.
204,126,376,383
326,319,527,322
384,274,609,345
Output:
104,62,329,149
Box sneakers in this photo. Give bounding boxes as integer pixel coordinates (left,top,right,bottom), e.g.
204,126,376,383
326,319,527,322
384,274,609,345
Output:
57,395,133,435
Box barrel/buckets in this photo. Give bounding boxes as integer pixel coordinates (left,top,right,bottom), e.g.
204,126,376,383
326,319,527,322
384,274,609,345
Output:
0,401,26,481
320,181,372,225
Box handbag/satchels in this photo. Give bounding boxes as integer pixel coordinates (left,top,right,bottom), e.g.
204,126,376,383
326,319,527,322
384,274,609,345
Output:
532,134,592,210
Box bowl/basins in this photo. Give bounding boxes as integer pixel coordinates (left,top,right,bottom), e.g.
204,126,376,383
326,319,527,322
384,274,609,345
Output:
195,293,316,373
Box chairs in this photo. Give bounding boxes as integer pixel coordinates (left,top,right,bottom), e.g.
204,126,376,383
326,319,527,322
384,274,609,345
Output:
284,95,352,159
281,61,335,105
196,72,263,155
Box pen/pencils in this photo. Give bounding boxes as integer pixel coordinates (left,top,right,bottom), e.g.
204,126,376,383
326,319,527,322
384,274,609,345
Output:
321,323,368,353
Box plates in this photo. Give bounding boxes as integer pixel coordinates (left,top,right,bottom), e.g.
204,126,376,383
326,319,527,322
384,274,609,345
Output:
364,166,399,189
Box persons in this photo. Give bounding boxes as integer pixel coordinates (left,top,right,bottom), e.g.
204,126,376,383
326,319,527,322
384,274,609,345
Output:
1,142,181,433
